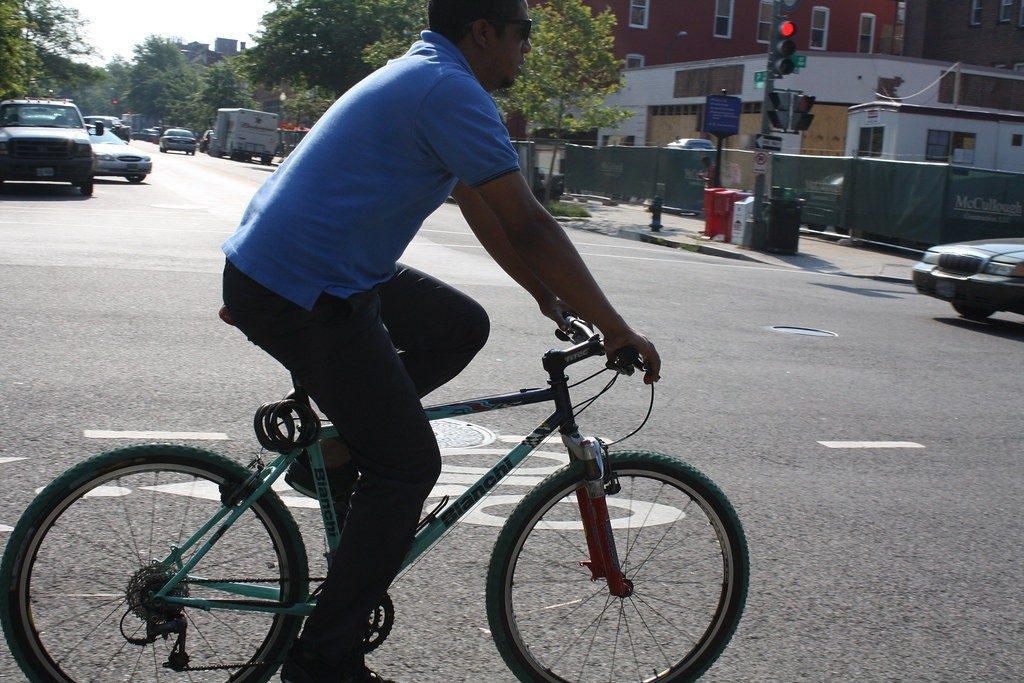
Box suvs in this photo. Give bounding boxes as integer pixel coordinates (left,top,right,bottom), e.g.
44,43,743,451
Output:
0,99,104,194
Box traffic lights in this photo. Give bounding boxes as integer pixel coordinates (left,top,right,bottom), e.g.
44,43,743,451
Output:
772,14,796,74
767,89,790,129
112,100,118,104
790,92,816,130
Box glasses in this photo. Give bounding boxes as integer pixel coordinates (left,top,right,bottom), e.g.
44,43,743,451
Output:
484,15,532,42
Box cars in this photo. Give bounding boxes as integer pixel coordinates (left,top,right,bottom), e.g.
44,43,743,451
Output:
912,237,1024,320
69,113,199,183
199,130,213,152
159,129,196,155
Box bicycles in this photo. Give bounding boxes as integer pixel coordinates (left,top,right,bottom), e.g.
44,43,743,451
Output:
1,305,749,683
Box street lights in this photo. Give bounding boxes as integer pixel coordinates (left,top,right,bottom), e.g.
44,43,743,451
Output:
279,74,301,149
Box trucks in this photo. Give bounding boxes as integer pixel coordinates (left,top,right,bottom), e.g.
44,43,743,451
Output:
207,108,278,164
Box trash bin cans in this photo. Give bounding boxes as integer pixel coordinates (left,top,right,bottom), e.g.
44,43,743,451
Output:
767,198,805,255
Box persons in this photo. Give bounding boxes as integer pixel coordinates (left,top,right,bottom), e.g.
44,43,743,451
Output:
221,1,662,683
698,156,716,233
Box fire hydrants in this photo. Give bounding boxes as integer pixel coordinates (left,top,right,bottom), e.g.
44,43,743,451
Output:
649,194,664,232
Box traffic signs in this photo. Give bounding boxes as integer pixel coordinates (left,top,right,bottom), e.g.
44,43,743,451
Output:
756,132,783,152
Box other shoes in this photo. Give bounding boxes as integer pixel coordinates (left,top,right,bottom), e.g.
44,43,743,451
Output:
280,637,399,683
285,449,318,504
